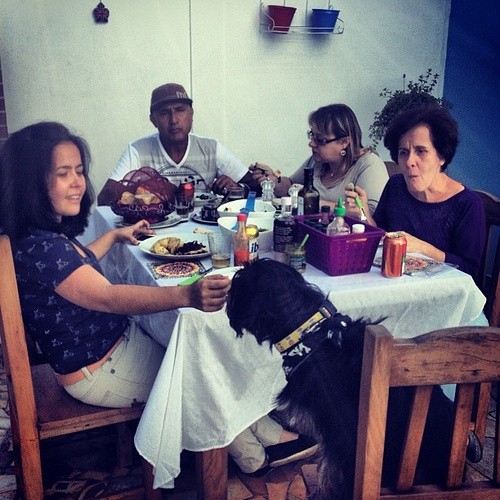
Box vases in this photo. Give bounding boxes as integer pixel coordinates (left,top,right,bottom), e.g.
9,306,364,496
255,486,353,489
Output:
268,4,297,34
312,8,340,34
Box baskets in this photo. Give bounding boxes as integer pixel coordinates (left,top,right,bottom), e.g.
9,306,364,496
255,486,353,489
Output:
295,213,385,275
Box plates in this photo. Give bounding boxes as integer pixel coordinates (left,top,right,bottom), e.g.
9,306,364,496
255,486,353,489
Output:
191,211,218,224
114,213,181,228
139,234,212,258
204,266,245,296
193,192,225,208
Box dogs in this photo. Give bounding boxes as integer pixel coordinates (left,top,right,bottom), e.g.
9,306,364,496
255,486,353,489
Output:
222,254,467,500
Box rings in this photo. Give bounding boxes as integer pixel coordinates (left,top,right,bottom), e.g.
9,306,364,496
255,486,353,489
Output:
224,187,228,194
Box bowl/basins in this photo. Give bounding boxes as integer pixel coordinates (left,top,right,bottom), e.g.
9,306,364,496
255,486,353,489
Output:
217,216,274,253
217,200,276,217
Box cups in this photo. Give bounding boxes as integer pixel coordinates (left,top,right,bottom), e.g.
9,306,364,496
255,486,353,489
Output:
207,232,231,269
175,195,189,222
227,187,244,202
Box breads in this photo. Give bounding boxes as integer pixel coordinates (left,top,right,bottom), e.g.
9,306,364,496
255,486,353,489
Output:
118,185,165,205
149,237,182,255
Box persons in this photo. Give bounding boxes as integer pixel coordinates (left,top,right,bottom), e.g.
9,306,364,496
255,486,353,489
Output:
253,104,390,218
345,103,486,463
1,121,320,480
96,84,261,206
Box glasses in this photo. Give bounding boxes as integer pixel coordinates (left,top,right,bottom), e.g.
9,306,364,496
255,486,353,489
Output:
308,130,347,147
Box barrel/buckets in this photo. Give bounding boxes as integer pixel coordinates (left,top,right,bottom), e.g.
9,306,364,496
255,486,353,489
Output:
268,5,297,34
312,9,340,31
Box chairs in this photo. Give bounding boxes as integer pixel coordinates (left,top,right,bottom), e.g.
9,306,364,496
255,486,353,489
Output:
353,323,500,500
0,236,162,500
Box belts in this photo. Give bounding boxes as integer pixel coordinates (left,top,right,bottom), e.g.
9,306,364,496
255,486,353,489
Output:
54,335,124,386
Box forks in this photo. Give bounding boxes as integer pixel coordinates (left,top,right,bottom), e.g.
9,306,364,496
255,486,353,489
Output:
253,164,283,180
349,183,368,224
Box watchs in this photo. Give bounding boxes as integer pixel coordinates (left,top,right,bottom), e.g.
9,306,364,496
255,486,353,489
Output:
238,183,250,198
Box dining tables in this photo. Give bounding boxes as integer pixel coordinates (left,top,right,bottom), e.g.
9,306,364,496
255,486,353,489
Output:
89,206,487,500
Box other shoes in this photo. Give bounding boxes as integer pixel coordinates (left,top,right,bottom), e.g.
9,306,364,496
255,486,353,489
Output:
466,432,481,462
252,462,273,480
266,435,320,468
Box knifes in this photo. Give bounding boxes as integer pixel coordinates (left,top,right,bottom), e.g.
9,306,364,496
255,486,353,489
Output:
177,268,212,286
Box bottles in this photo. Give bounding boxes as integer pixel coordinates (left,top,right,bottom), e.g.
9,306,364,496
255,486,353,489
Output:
296,167,320,216
320,206,330,225
326,197,350,236
285,244,305,273
352,224,364,234
273,197,298,252
233,214,249,266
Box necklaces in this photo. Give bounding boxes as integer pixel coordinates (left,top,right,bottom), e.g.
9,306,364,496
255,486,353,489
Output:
331,164,342,178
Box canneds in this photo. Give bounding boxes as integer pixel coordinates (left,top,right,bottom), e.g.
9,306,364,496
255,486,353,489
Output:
381,231,407,278
283,241,307,273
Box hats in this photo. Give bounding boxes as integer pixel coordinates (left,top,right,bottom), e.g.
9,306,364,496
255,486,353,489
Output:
149,84,192,114
